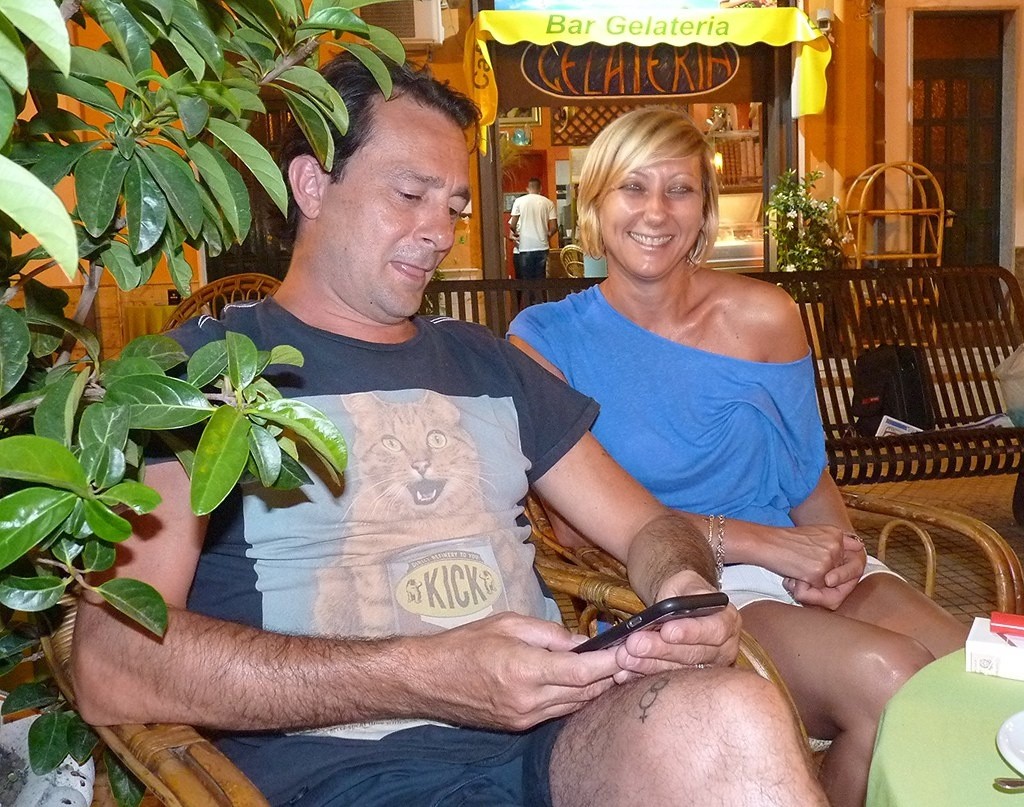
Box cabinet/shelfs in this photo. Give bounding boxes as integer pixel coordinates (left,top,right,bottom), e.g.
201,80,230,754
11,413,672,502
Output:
838,160,954,358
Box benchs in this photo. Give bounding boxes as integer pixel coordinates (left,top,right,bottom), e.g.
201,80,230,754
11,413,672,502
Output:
364,261,1024,522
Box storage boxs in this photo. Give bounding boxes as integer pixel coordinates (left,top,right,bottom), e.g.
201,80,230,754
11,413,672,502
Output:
964,617,1024,681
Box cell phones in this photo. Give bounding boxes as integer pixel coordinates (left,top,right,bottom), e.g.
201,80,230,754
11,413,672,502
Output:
570,594,730,654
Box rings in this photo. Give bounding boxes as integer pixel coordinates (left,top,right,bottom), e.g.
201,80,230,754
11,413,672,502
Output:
694,664,704,670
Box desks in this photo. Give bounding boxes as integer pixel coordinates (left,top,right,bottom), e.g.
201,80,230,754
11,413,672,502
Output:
866,648,1024,807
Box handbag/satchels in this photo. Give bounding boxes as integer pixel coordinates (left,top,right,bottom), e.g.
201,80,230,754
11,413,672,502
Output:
850,344,935,437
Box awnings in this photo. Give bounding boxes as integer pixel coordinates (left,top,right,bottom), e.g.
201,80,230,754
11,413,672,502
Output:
463,8,833,157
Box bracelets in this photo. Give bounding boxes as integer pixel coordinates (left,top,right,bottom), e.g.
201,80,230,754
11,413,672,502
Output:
847,534,867,566
703,514,726,581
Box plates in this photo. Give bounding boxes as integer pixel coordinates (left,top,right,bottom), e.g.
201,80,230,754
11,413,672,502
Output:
995,709,1024,776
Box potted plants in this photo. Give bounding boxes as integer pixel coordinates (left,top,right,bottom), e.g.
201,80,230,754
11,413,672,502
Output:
762,170,854,359
0,1,404,807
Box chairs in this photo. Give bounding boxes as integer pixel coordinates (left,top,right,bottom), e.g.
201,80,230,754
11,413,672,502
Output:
30,406,1024,807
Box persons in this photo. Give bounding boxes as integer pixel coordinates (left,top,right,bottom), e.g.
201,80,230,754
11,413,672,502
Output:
506,107,970,807
68,51,831,807
